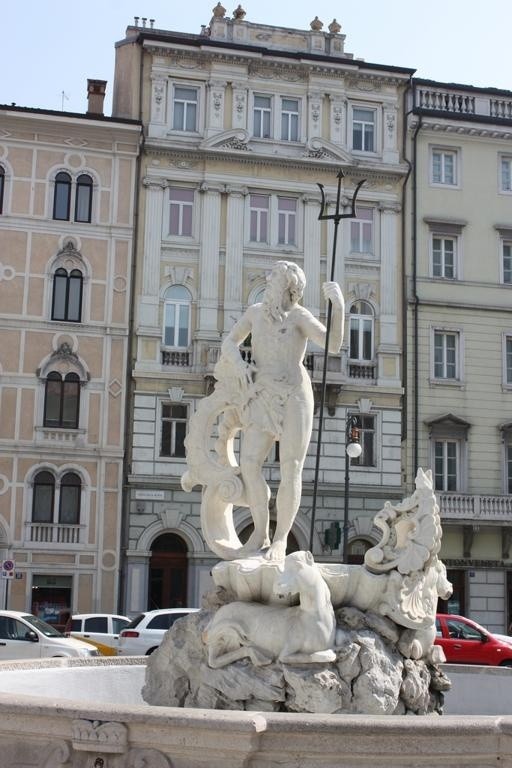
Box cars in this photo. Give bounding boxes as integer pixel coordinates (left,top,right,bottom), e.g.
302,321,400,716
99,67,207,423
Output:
65,611,134,647
0,609,100,661
434,612,512,665
118,607,200,655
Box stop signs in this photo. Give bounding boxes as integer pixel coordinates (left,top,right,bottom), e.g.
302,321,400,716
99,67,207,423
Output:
2,560,15,579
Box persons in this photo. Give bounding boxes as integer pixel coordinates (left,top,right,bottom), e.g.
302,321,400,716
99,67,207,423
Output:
218,259,347,565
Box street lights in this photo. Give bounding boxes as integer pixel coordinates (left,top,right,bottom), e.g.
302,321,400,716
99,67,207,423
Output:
343,418,364,562
